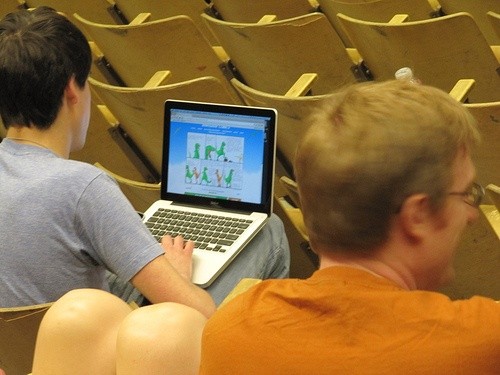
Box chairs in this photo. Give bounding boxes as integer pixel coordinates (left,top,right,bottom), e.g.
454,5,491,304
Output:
0,0,500,375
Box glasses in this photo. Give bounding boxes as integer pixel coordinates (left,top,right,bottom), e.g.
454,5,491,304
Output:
427,183,484,207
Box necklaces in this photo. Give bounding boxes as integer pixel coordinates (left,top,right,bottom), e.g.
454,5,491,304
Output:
7,138,48,150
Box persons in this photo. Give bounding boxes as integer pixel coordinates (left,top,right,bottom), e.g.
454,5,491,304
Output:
0,6,217,321
191,81,500,375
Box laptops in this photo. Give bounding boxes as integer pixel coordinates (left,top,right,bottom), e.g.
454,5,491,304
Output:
139,98,278,289
